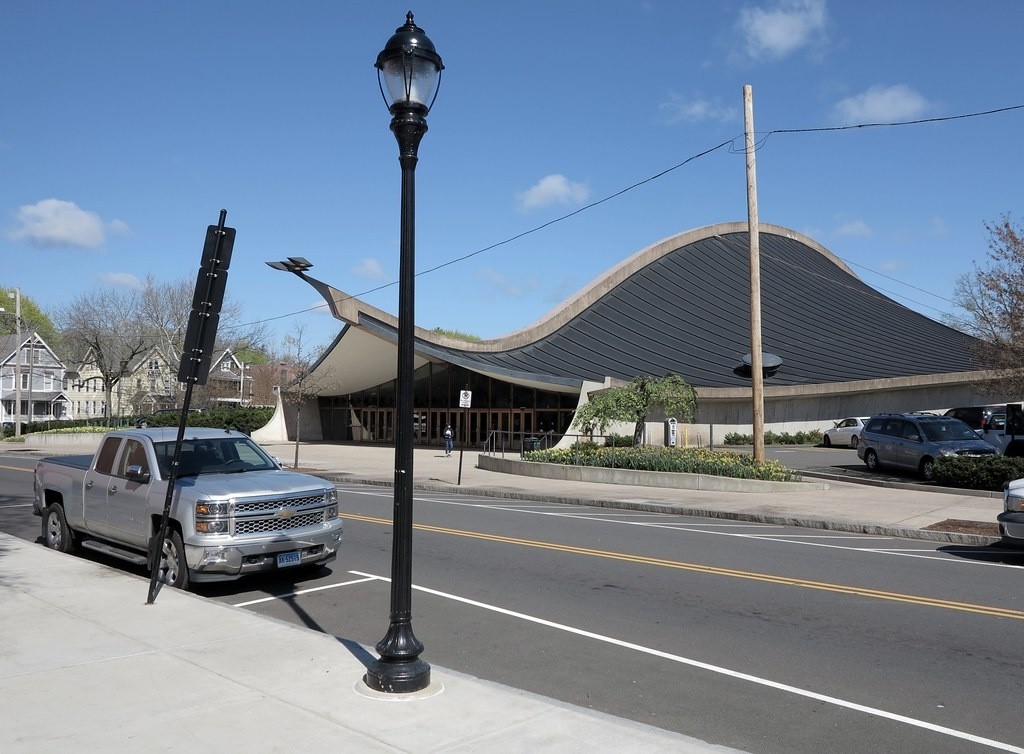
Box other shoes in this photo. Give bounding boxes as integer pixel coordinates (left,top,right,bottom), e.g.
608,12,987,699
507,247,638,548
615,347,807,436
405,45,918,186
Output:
446,455,449,458
448,454,451,458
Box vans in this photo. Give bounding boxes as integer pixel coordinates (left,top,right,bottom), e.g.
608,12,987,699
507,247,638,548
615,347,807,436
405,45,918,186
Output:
943,402,1024,458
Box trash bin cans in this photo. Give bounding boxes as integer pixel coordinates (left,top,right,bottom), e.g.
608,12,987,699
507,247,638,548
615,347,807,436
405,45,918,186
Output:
523,437,540,450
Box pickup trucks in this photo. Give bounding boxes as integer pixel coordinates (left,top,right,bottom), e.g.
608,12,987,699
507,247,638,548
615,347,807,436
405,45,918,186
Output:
32,427,344,593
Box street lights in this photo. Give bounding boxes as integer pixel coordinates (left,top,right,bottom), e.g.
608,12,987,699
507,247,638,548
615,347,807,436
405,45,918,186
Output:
0,307,21,439
365,10,447,694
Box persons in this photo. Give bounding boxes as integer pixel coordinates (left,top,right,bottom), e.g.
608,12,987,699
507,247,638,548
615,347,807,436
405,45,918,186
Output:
442,423,454,458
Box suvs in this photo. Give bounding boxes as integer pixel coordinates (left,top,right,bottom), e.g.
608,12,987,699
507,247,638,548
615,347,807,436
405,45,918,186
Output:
857,411,1000,481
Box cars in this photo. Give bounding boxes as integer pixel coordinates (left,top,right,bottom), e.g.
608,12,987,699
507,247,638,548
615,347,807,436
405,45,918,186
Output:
997,478,1024,541
823,416,871,450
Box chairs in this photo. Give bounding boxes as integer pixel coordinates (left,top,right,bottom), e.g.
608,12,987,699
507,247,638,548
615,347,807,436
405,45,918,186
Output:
193,443,223,466
